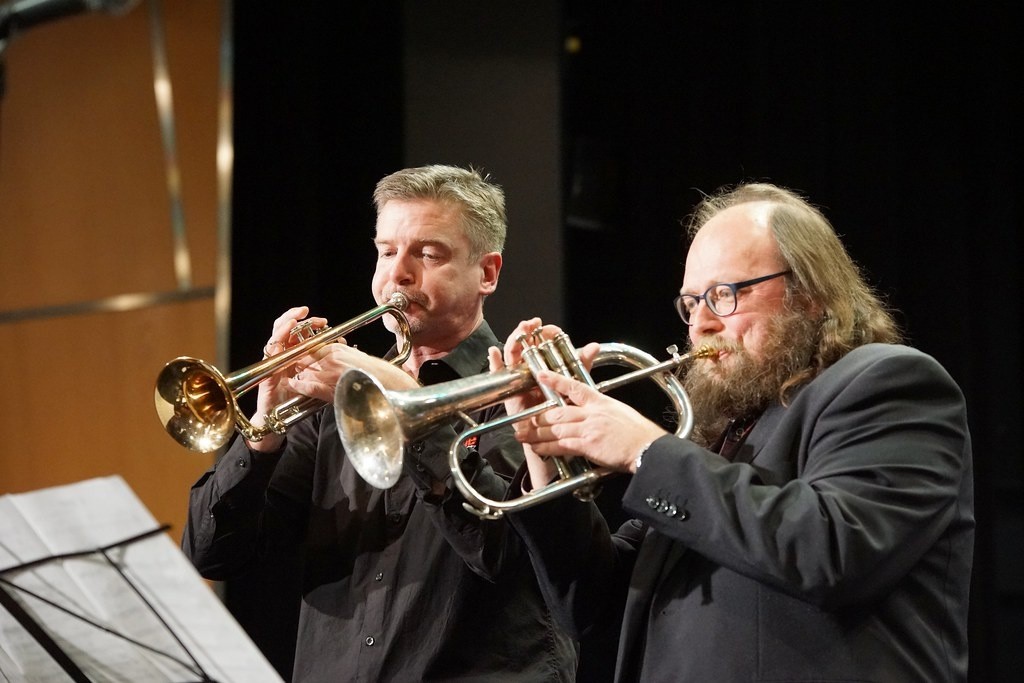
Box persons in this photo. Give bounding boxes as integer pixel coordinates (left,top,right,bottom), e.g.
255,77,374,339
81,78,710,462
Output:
487,182,976,683
181,162,580,683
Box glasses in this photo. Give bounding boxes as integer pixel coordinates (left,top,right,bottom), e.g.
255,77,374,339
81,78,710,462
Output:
674,268,794,326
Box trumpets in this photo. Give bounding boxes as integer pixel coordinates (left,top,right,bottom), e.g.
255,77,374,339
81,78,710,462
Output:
153,291,414,455
332,324,719,521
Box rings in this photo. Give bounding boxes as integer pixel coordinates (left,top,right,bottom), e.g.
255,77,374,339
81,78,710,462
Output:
293,374,299,380
